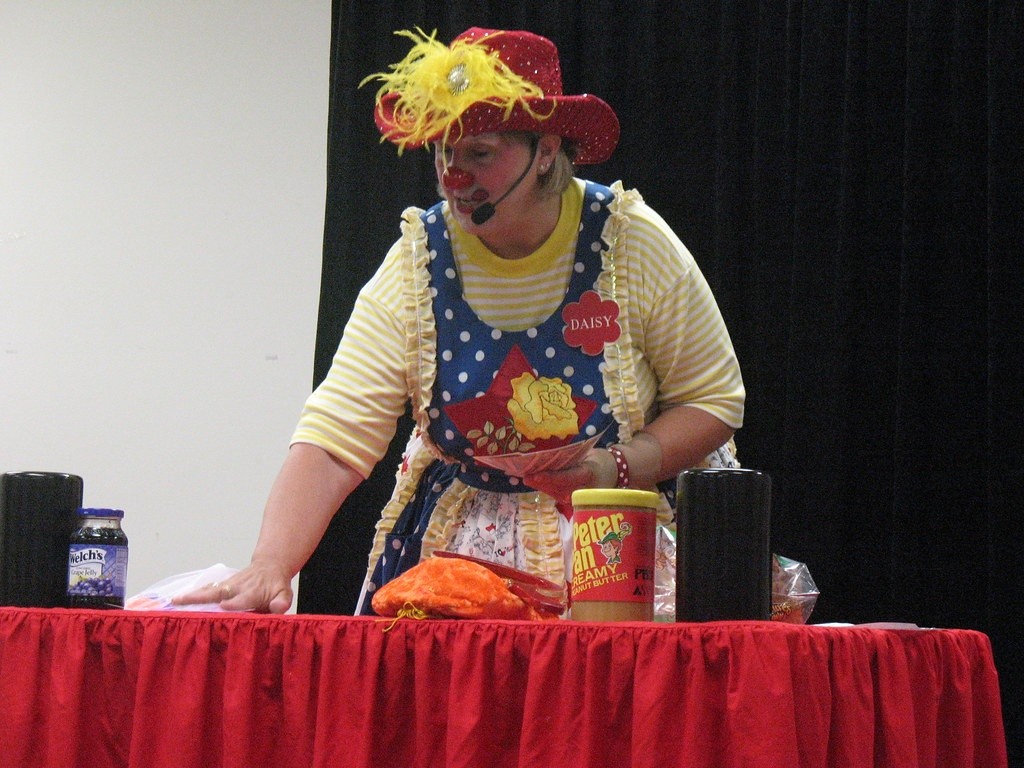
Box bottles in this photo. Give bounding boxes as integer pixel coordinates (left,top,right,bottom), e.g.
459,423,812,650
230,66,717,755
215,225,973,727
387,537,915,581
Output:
571,488,660,621
675,468,772,621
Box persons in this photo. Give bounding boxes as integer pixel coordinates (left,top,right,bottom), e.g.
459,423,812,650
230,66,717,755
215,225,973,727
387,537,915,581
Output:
172,26,747,615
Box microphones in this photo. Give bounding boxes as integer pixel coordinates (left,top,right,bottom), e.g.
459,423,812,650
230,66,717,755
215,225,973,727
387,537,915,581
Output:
471,138,540,225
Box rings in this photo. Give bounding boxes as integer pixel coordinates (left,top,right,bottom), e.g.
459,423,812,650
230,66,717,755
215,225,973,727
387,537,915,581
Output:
225,585,232,597
211,582,219,588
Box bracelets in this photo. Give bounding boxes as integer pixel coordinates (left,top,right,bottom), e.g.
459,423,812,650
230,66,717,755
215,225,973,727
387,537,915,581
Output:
606,447,629,489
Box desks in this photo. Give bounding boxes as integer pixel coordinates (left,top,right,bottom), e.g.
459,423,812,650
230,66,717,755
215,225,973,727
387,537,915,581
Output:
0,605,1008,768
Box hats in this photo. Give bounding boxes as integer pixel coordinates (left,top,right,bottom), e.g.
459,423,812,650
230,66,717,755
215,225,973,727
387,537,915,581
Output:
358,26,620,165
